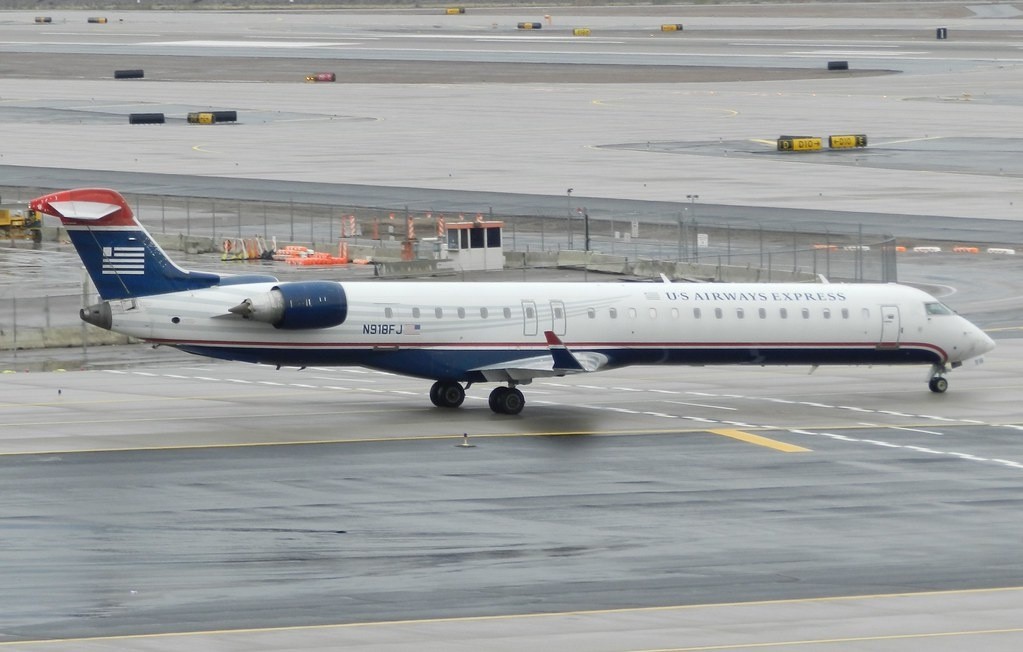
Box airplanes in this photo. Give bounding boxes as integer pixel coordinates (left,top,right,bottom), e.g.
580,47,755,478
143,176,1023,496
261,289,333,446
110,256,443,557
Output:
26,186,997,419
